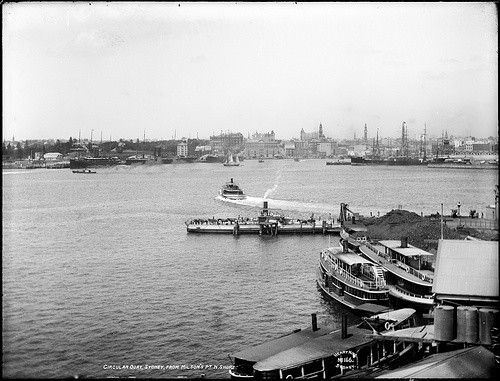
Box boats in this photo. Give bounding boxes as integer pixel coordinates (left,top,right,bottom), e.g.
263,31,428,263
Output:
340,215,368,249
326,159,351,164
226,307,417,380
221,177,245,198
317,240,392,313
223,159,240,165
359,235,435,310
72,168,96,172
